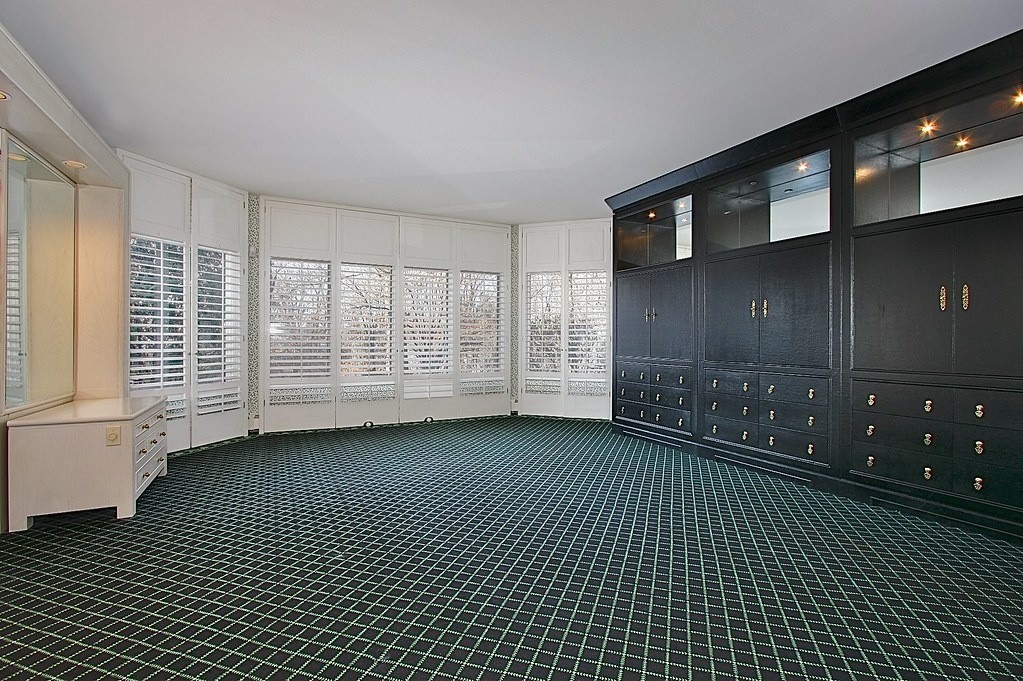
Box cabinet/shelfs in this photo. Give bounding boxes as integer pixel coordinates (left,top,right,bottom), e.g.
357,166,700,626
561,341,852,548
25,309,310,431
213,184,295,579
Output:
5,394,169,534
602,27,1023,543
